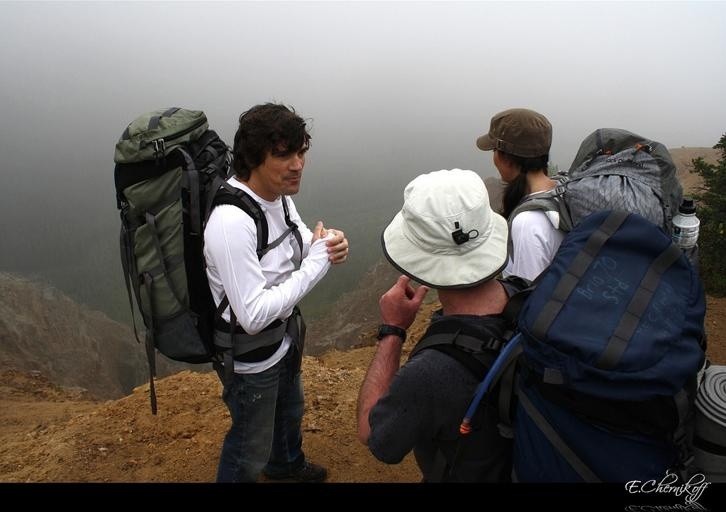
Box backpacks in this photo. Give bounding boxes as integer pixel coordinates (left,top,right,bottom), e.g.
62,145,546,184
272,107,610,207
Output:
410,210,705,483
506,128,699,265
115,107,303,363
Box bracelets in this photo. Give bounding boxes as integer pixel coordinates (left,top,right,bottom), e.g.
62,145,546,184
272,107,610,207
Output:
375,320,406,343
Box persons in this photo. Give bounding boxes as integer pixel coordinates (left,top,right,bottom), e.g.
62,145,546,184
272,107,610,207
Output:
201,101,350,482
350,169,537,485
476,109,567,284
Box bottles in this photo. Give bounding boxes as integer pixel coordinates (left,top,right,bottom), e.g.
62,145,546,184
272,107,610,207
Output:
670,196,699,259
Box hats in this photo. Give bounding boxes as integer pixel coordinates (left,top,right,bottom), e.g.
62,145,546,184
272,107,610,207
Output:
476,108,552,157
381,168,509,290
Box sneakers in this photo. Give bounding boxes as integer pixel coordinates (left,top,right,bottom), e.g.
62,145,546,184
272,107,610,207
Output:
265,461,326,481
